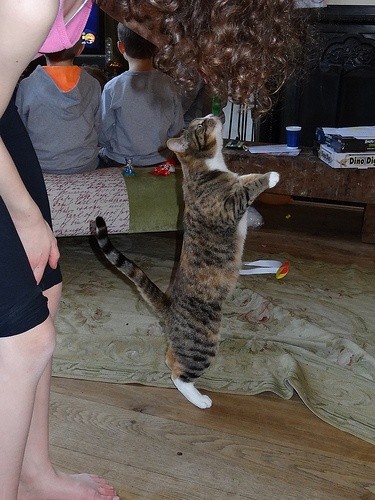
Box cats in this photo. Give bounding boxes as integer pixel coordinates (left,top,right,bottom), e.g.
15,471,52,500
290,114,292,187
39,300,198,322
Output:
95,113,282,411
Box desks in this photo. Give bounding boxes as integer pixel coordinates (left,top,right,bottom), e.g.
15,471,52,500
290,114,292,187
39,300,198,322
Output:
223,138,375,244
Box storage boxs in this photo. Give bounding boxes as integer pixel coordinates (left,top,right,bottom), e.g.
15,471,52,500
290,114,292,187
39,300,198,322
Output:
319,126,375,170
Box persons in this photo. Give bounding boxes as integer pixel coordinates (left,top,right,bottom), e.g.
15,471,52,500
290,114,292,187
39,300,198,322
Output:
123,157,135,176
0,0,300,500
148,159,174,177
93,22,185,168
16,32,102,175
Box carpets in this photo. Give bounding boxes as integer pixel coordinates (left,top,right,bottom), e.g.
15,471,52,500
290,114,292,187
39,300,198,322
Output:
45,241,375,446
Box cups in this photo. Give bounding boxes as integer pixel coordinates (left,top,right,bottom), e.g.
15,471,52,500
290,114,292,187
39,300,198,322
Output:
285,126,302,149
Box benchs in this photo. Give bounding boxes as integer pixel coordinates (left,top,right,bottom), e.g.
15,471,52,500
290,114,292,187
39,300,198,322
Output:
42,167,190,236
